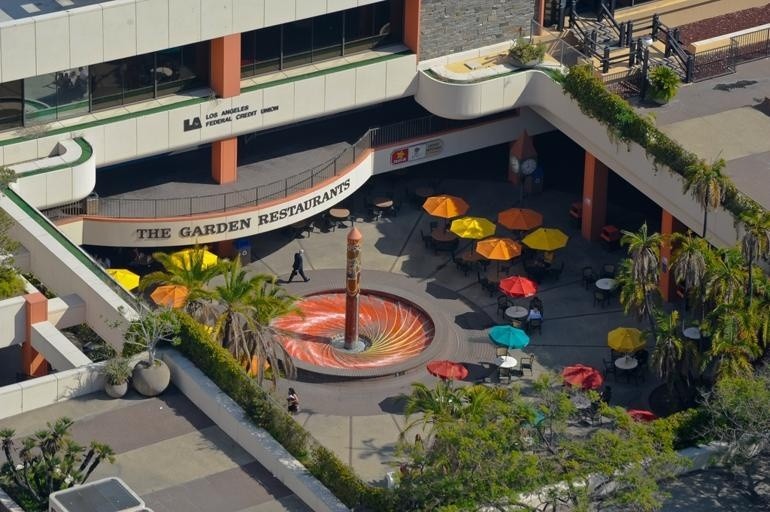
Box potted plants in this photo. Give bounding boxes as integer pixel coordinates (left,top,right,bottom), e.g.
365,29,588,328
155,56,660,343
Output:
508,32,546,67
99,355,136,398
645,64,684,105
104,294,185,397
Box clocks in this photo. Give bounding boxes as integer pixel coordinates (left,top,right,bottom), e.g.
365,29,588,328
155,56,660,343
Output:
508,156,538,177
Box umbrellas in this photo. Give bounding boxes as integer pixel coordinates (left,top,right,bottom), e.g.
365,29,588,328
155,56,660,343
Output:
423,195,470,219
171,250,218,273
106,268,140,291
608,327,647,354
498,276,538,299
450,208,569,262
560,363,604,390
426,360,470,380
151,285,189,309
489,325,531,350
627,408,657,425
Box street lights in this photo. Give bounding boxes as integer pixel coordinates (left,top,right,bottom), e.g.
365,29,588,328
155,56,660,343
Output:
638,35,652,102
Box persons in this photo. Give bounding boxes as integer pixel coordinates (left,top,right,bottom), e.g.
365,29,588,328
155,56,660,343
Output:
287,249,311,283
287,388,300,416
52,66,88,102
98,256,111,268
601,383,612,405
526,298,544,336
541,251,554,269
633,348,649,368
413,433,425,452
